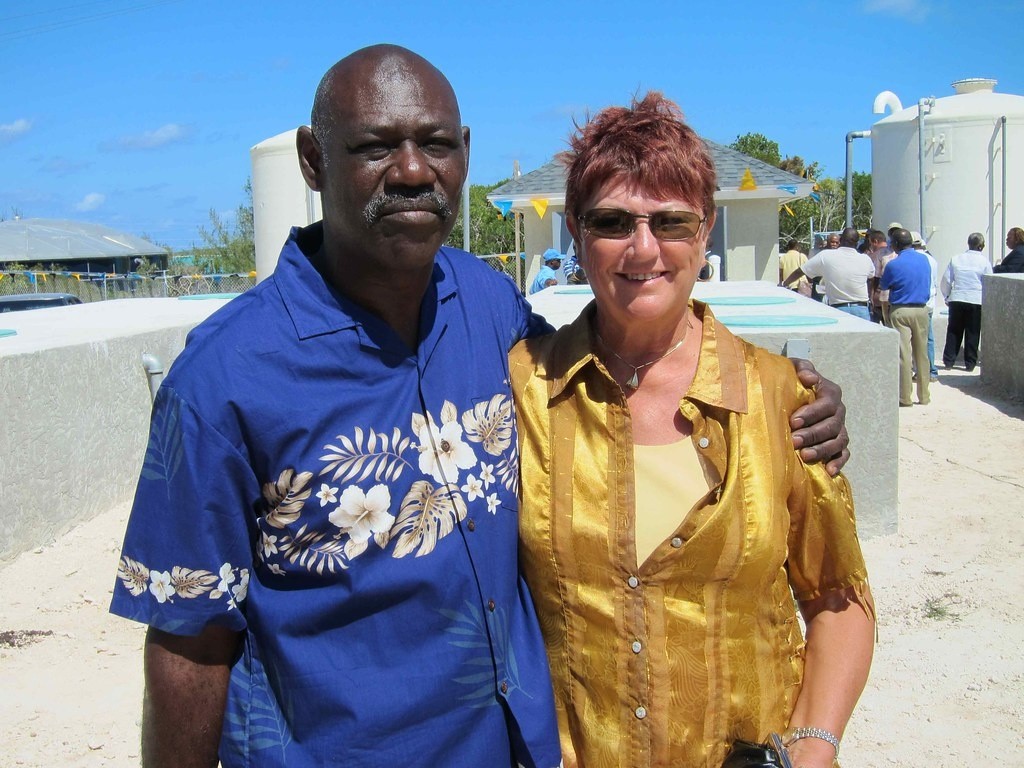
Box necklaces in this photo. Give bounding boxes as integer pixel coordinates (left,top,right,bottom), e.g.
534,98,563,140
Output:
597,330,686,389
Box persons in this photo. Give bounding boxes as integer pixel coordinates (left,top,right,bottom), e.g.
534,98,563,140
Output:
993,227,1024,273
857,223,939,382
880,229,932,407
940,233,993,372
530,248,566,295
815,234,839,302
779,239,812,292
778,228,875,320
563,241,589,285
506,94,876,768
110,43,850,768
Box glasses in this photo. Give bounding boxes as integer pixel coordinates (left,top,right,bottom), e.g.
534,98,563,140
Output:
576,208,708,240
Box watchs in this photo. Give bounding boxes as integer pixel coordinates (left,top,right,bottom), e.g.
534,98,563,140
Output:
782,727,839,757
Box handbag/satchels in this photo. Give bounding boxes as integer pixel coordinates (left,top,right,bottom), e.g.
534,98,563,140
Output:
719,738,784,768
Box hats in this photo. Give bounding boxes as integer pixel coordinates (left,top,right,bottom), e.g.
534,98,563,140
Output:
887,223,902,231
910,232,921,244
921,239,926,247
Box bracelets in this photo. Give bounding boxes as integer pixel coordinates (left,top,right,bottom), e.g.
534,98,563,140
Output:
782,281,784,286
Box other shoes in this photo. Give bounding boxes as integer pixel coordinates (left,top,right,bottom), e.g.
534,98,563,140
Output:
912,374,938,383
944,365,953,370
920,398,931,405
966,367,973,372
899,401,913,407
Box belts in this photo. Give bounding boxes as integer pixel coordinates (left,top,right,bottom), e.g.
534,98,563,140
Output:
831,302,870,308
